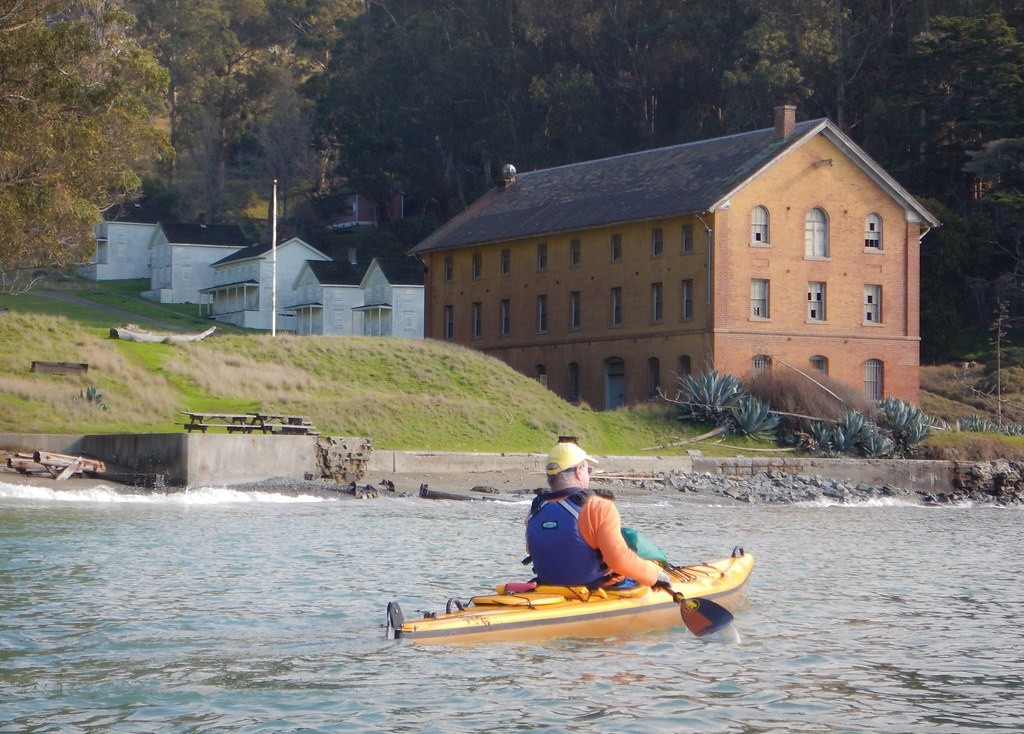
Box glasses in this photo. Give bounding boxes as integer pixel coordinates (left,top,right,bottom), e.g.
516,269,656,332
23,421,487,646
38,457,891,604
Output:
582,464,593,474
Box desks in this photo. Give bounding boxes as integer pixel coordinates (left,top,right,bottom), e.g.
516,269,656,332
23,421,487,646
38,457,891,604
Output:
246,412,310,434
180,411,256,434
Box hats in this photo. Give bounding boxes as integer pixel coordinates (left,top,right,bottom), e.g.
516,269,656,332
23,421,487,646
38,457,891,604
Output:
545,442,599,476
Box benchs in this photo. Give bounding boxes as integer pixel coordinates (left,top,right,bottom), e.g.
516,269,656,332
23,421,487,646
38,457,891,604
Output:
262,423,314,428
175,422,192,425
196,423,261,428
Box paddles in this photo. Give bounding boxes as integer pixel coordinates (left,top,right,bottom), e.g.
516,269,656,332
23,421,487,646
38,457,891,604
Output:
658,580,734,639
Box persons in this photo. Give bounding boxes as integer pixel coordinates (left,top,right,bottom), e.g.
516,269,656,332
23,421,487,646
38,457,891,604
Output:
524,442,671,587
